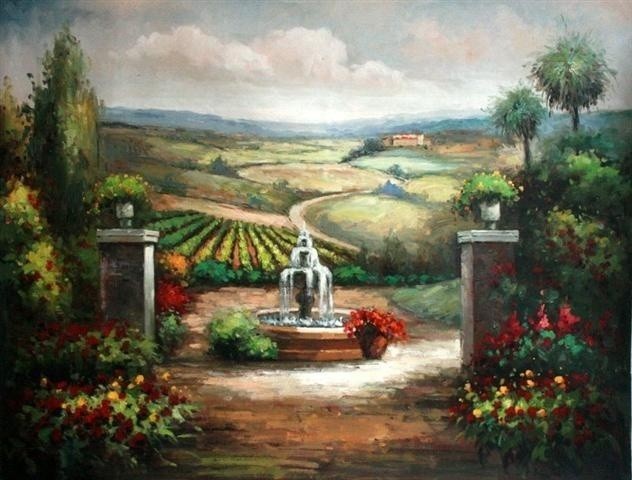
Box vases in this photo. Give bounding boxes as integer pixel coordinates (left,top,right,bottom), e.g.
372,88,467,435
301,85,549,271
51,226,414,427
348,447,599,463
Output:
114,200,136,227
478,197,501,229
358,333,388,360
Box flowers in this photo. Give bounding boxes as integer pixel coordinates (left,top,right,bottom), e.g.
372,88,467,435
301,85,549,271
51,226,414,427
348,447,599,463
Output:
94,169,150,214
342,304,407,348
446,170,521,224
